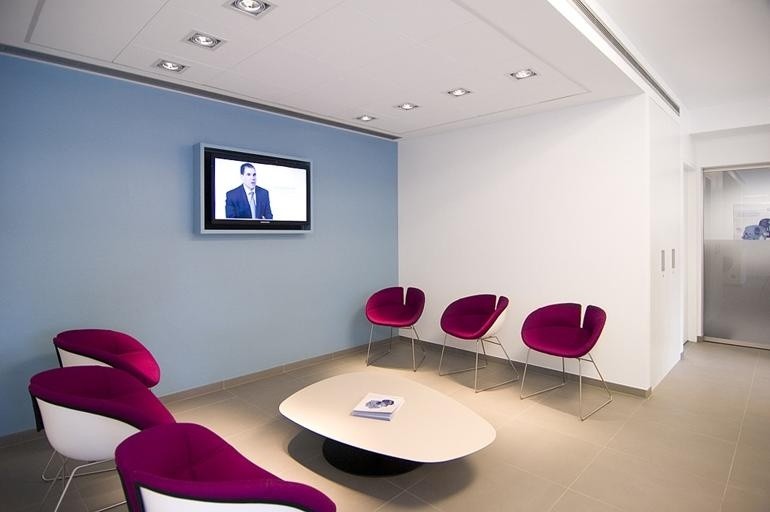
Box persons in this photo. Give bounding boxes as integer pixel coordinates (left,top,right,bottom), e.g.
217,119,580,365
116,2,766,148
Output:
226,163,273,221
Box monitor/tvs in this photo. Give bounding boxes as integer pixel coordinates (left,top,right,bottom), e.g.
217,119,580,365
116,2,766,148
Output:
194,141,313,235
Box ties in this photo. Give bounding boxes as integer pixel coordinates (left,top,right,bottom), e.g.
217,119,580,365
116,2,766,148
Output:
250,192,256,219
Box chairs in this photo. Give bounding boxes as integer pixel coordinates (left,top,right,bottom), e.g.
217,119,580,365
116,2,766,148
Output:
29,366,176,512
41,330,160,483
520,303,613,420
365,287,427,372
115,423,336,511
438,295,520,393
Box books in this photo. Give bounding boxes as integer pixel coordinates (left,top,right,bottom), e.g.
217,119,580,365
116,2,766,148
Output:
352,392,405,420
